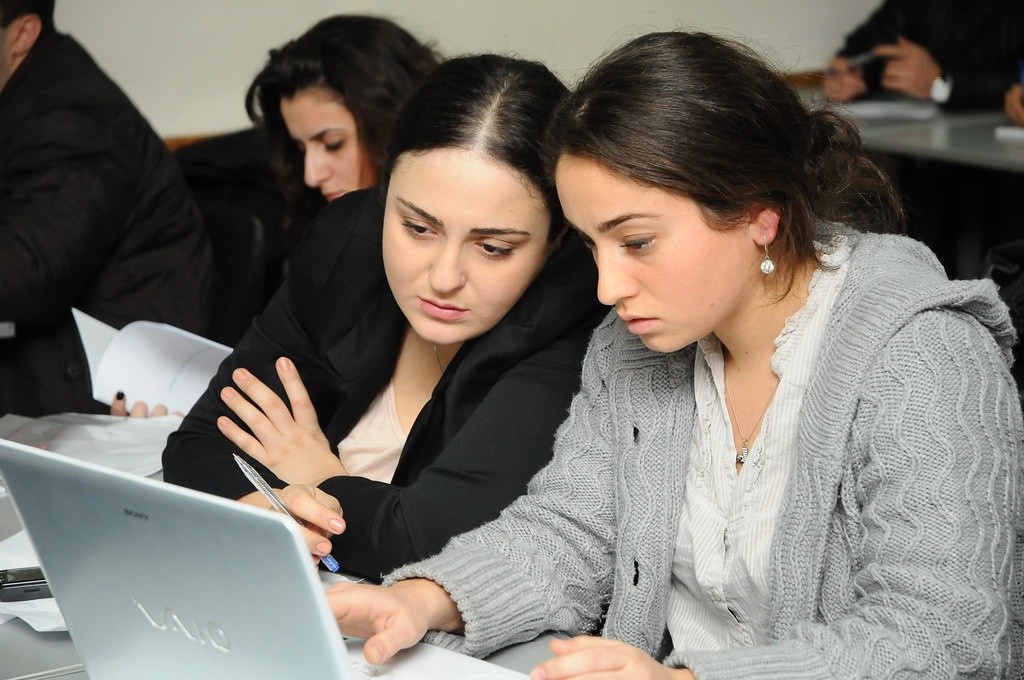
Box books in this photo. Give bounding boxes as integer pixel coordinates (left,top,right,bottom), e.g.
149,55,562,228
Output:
71,307,234,418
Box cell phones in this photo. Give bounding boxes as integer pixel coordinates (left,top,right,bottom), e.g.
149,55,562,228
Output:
0,567,57,603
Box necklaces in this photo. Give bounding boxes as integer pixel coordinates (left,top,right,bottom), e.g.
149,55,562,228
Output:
434,344,443,374
725,355,780,463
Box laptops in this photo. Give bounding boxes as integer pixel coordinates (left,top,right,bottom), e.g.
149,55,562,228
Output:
0,438,536,680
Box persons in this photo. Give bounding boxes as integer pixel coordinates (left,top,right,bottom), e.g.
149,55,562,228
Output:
328,32,1023,680
162,54,614,584
2,0,437,419
823,0,1024,400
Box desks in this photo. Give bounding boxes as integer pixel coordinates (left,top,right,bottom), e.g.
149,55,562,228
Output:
833,114,1024,279
0,413,559,680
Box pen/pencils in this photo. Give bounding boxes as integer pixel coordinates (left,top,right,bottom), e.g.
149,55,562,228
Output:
230,452,340,573
825,48,878,82
